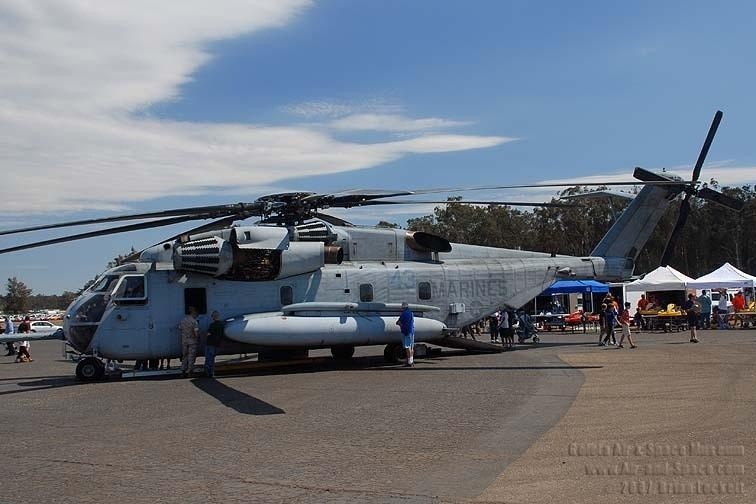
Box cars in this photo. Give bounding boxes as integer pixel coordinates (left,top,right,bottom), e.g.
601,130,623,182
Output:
0,312,65,323
25,321,62,336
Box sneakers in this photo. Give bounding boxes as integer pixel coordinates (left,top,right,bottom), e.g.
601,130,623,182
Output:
403,363,415,368
598,339,639,351
491,340,516,349
690,338,699,344
181,370,196,378
207,373,214,377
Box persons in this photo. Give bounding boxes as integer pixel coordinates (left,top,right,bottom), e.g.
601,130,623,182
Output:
202,310,225,378
462,288,755,349
127,280,144,297
396,302,415,367
181,307,200,378
0,313,48,362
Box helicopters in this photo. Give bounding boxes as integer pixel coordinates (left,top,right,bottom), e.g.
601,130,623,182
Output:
7,109,746,382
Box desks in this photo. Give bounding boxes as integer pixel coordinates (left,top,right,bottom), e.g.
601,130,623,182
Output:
530,310,756,340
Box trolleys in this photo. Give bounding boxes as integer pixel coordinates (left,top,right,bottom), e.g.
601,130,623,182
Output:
512,318,540,344
